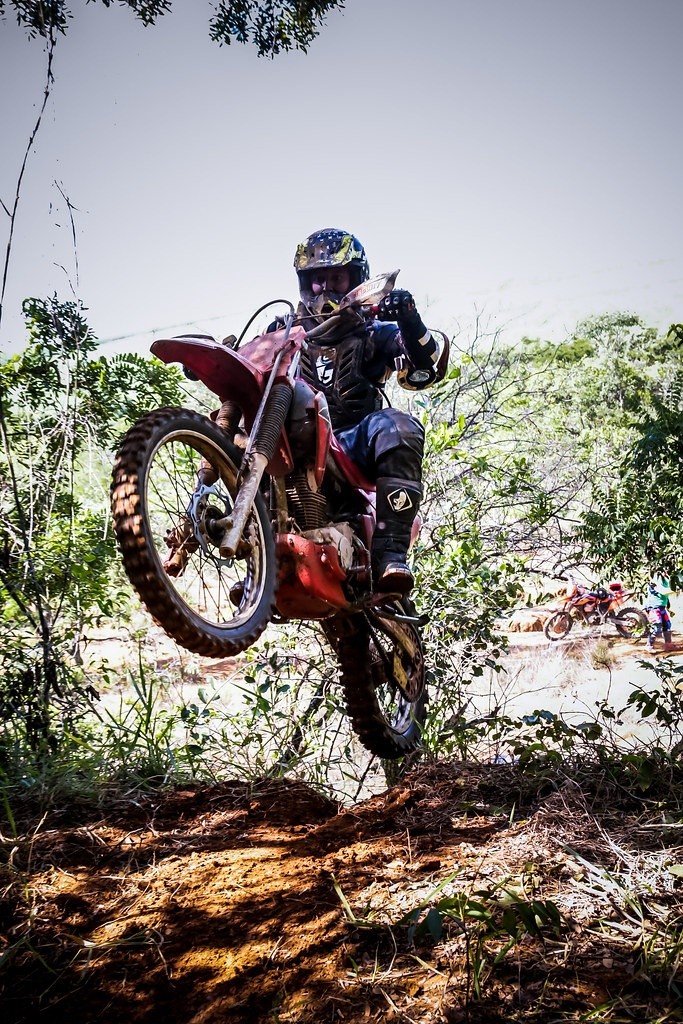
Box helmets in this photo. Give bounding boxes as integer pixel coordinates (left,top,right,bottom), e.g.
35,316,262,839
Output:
294,228,370,320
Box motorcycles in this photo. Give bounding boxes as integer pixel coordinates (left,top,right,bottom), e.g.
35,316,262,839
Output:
541,574,650,643
108,268,432,758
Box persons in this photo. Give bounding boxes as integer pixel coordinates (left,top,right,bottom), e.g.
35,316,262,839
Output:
645,572,675,652
240,226,445,598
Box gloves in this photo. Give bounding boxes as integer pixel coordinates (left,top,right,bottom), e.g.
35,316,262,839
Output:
376,291,427,346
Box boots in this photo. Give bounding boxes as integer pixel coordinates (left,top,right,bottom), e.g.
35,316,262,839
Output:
663,631,683,650
644,635,657,654
371,476,424,593
230,582,247,607
